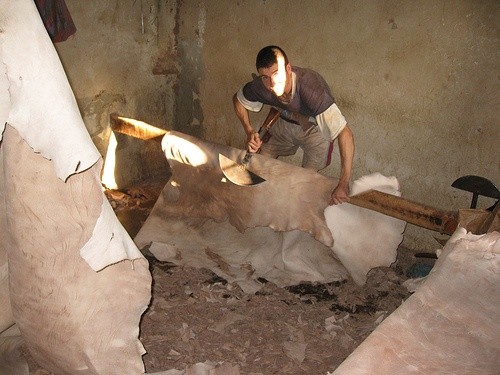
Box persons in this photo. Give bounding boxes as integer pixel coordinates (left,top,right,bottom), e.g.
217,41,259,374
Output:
231,45,356,205
